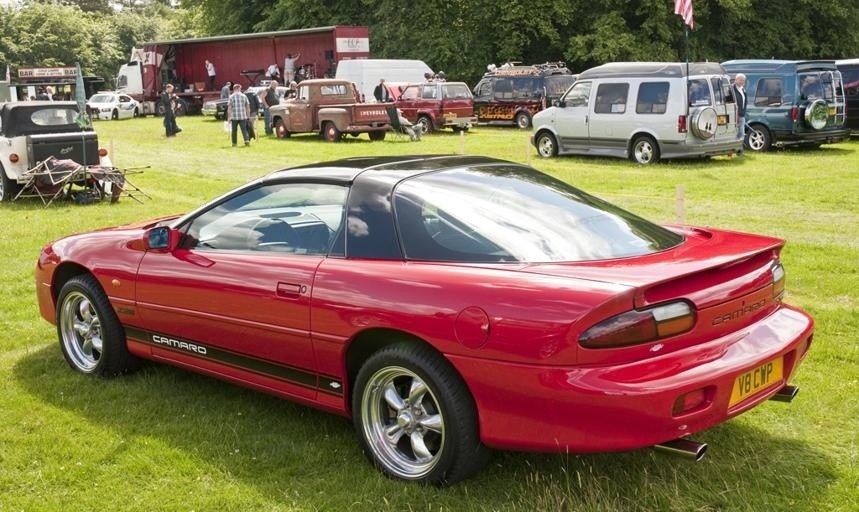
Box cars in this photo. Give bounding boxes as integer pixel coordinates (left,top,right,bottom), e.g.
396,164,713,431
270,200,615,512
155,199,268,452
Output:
202,85,304,116
85,91,140,120
0,99,113,202
34,153,819,485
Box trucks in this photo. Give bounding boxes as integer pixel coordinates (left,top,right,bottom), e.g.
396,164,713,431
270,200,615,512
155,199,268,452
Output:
114,24,371,116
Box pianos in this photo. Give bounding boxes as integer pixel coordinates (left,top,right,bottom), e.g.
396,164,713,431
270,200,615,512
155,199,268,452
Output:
240,69,264,76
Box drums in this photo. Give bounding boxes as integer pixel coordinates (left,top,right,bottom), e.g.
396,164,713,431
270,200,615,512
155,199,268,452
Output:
298,66,306,75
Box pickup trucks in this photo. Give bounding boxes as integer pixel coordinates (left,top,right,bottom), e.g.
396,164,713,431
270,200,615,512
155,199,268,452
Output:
268,78,391,141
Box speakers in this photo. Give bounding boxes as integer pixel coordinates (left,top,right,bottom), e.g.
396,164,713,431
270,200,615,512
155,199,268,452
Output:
325,50,333,59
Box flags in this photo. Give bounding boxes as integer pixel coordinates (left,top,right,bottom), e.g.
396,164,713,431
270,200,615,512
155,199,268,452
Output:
673,0,694,31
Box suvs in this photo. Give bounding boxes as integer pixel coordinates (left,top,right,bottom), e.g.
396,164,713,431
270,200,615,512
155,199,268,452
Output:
386,71,474,135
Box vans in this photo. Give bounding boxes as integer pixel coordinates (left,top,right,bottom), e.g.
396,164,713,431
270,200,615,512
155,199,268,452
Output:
716,59,852,153
334,59,438,103
833,58,859,138
531,59,743,164
472,60,577,128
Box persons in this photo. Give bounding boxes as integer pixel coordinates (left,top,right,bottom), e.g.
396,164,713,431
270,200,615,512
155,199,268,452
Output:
227,84,251,147
731,73,748,156
205,59,216,91
22,86,71,101
161,83,182,137
85,100,92,123
424,70,448,99
284,81,298,101
265,64,280,84
374,79,389,103
243,88,260,141
220,81,231,99
260,80,280,136
283,53,301,87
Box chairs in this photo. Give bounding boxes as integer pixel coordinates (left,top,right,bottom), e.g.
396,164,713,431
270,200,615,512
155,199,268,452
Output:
386,103,420,141
